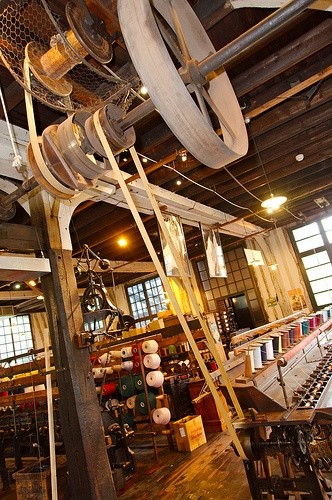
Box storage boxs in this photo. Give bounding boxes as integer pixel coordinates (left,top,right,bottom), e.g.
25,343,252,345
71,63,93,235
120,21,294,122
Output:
12,454,70,500
173,415,208,453
162,313,189,328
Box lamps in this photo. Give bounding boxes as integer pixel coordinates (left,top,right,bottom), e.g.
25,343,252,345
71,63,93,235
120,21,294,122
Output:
246,124,287,212
